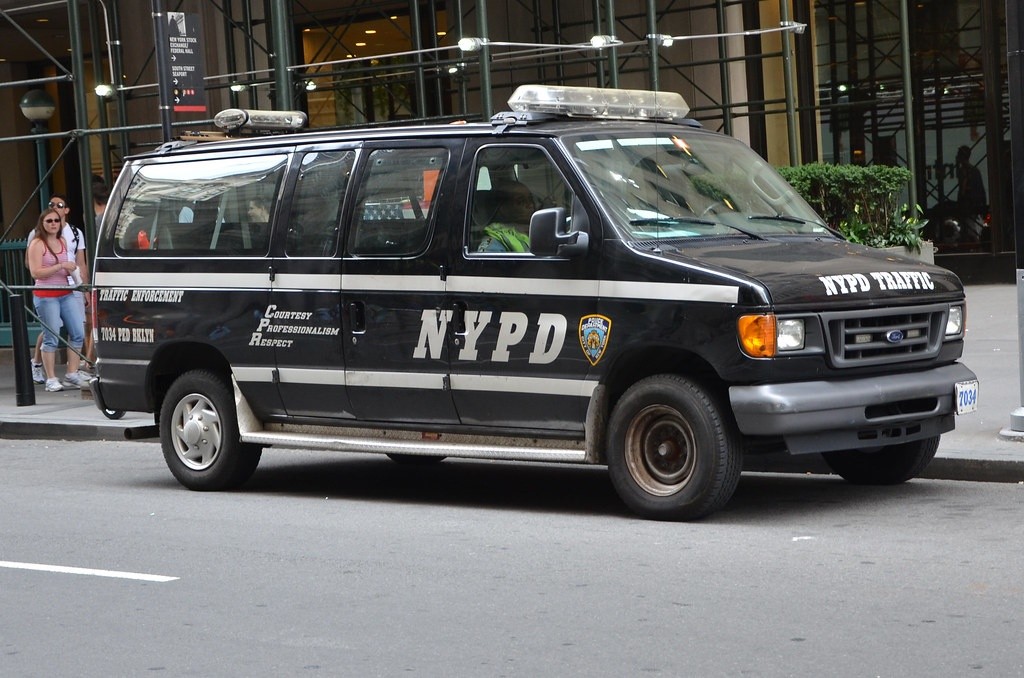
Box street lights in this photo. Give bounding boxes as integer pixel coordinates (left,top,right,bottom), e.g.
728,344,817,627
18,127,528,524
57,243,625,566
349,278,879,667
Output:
19,89,58,215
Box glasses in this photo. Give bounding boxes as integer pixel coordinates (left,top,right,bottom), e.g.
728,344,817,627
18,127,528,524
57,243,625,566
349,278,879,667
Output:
48,201,66,208
43,219,61,223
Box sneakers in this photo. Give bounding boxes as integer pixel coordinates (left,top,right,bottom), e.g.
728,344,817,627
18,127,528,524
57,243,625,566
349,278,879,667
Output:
31,359,45,384
77,369,91,380
62,372,92,389
45,378,64,391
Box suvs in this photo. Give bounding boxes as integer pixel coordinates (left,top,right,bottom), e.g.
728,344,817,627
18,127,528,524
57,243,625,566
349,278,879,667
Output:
86,83,981,523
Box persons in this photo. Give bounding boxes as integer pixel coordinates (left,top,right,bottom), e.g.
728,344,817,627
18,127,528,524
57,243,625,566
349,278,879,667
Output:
92,183,109,236
958,145,983,241
28,209,85,392
477,181,534,253
25,193,91,383
248,197,268,222
